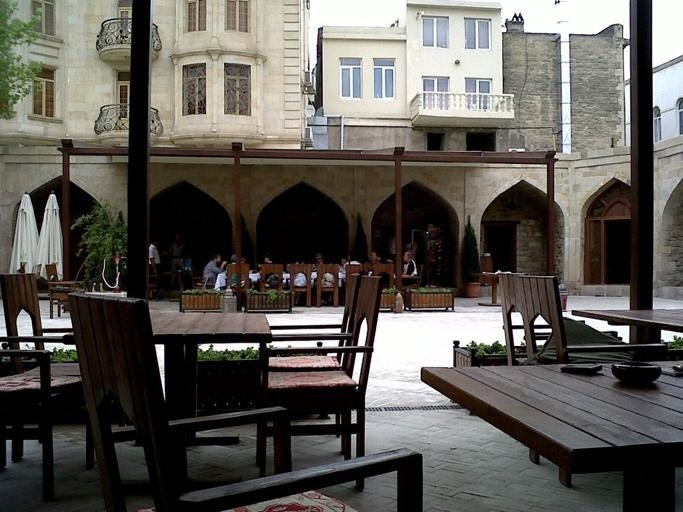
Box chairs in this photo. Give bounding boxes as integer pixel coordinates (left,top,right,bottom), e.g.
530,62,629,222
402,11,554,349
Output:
265,271,362,437
246,270,391,495
42,262,85,319
60,286,427,512
495,269,669,488
194,259,423,310
1,331,96,505
0,269,101,467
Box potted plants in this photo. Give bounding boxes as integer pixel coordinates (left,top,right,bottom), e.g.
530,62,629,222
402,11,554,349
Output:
459,220,483,299
176,287,225,313
404,284,456,312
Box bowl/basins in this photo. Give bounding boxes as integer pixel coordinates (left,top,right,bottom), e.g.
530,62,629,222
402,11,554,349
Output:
611,362,662,385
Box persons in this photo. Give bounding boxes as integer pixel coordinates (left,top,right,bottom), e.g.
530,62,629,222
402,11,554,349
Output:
69,236,89,280
146,230,417,307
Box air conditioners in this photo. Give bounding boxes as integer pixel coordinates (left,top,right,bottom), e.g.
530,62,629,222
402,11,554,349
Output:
507,148,526,152
302,69,315,94
303,126,314,149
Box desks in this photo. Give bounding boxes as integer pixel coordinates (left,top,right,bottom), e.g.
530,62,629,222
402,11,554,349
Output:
473,270,512,308
420,356,681,511
568,306,682,337
84,303,275,473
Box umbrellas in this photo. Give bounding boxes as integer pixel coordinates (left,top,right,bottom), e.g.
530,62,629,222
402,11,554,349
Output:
35,189,64,281
8,190,39,280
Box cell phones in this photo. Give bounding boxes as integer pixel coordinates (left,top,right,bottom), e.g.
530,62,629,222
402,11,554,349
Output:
561,364,603,373
